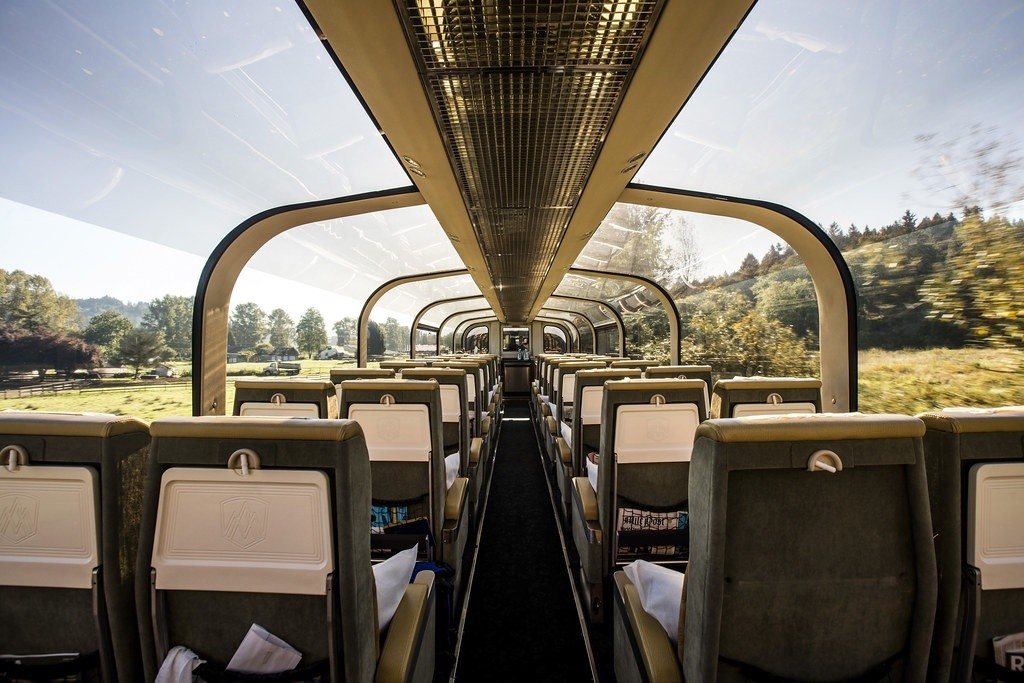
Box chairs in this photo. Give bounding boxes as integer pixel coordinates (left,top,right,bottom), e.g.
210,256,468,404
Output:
0,352,503,683
531,351,1024,683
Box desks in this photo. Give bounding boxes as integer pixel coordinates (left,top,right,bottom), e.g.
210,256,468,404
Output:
501,358,535,396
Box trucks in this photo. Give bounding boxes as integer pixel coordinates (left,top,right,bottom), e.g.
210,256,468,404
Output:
263,362,301,376
